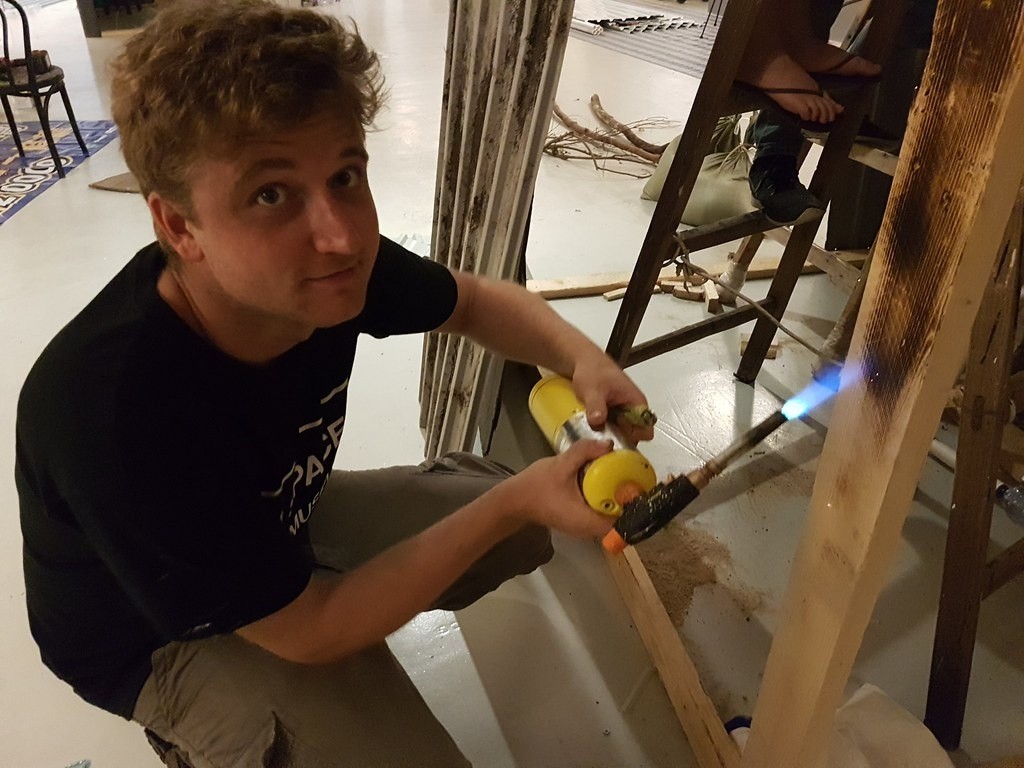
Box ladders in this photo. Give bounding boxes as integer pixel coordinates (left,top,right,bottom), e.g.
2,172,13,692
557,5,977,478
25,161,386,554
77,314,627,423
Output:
604,0,915,386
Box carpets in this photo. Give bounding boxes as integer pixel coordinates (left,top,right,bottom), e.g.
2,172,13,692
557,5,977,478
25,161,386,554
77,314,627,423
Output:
0,118,120,228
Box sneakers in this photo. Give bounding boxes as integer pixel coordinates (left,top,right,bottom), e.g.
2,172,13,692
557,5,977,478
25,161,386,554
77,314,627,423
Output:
749,161,825,226
802,121,900,152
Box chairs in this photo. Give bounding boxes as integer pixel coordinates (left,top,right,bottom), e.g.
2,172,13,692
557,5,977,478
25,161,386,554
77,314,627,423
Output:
0,0,89,180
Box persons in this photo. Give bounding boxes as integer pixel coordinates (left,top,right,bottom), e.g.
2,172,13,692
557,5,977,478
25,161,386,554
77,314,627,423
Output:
734,0,886,226
14,0,654,768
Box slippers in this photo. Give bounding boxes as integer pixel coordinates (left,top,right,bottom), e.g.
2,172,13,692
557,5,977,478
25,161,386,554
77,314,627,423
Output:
733,76,843,133
809,55,881,85
